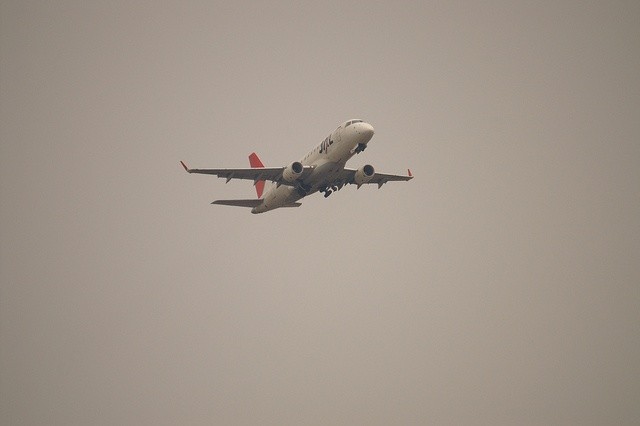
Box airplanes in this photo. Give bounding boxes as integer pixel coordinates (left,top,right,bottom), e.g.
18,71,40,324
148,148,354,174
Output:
180,118,413,213
